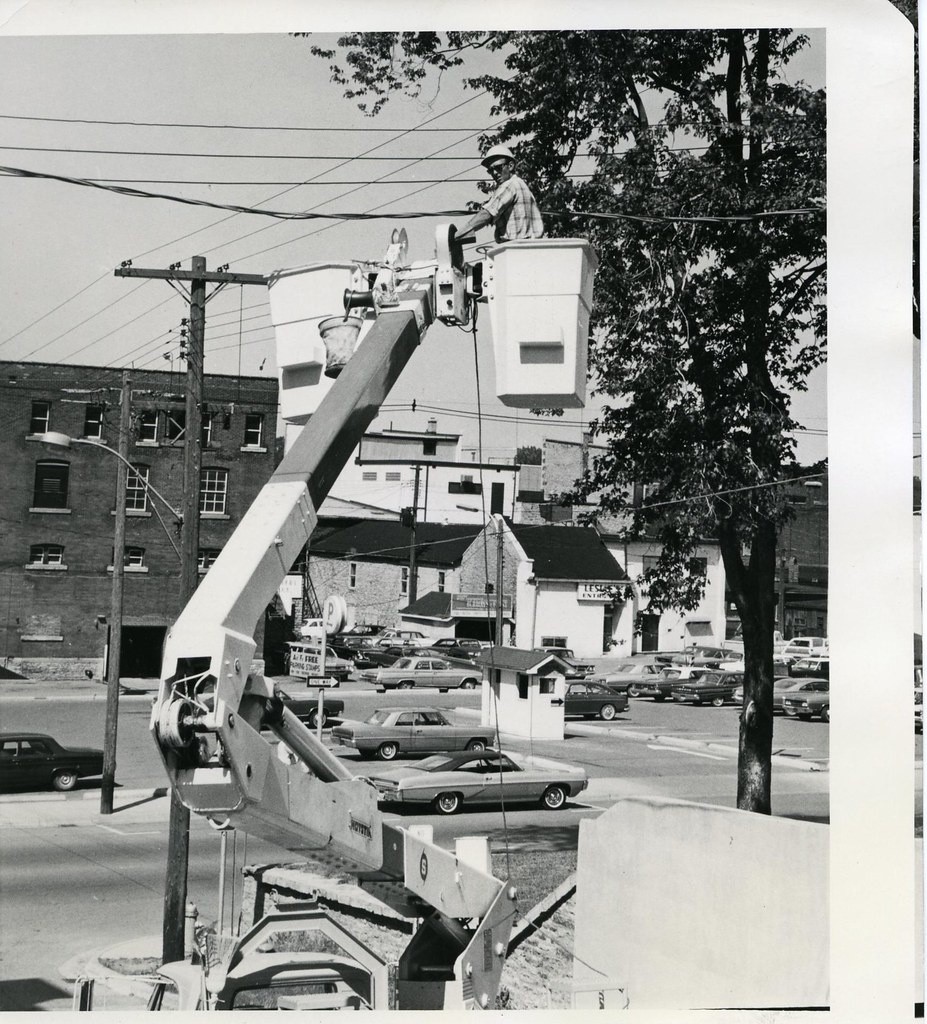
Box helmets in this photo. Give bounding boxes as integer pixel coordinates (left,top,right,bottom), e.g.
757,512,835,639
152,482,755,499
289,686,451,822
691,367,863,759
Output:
481,145,516,164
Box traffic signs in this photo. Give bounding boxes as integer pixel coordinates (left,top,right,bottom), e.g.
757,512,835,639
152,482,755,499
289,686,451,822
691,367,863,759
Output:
307,676,341,688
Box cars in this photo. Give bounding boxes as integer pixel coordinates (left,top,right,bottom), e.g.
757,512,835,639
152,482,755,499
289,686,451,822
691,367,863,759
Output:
301,617,333,640
0,732,117,791
289,644,355,680
531,647,595,680
732,676,794,705
369,750,588,814
791,658,829,674
381,631,432,648
915,692,923,731
565,680,629,721
719,651,790,673
372,638,424,655
337,625,388,642
360,657,483,692
584,661,678,698
428,638,484,661
782,681,830,723
635,667,718,702
330,706,497,759
671,671,745,707
276,690,345,729
369,646,433,669
773,678,829,716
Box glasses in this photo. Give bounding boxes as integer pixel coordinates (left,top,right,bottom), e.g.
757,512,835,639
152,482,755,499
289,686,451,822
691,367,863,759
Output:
487,162,508,174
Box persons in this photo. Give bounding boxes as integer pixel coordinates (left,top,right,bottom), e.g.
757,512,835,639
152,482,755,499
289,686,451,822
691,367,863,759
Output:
454,145,545,244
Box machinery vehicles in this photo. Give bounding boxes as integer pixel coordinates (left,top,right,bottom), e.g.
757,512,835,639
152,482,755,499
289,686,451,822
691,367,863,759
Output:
146,174,604,1009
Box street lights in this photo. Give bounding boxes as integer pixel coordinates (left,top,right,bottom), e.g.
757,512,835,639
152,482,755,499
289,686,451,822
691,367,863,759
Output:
27,431,202,964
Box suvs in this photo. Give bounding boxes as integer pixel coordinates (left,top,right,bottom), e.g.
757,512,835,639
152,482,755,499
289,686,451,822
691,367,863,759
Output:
673,646,734,668
784,637,824,662
340,638,383,668
